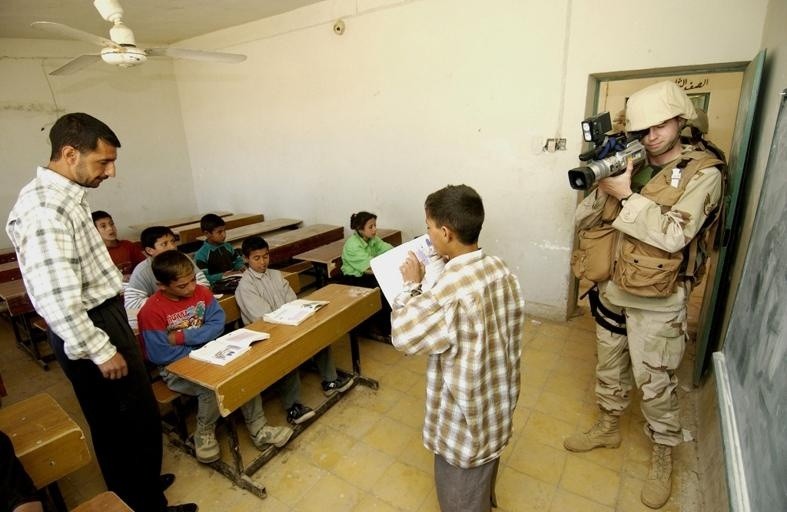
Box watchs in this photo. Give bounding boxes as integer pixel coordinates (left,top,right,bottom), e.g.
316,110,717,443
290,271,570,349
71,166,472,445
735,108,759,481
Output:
617,194,634,207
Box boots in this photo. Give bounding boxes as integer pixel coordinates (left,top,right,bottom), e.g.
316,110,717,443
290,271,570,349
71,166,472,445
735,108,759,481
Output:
641,443,673,509
563,407,621,452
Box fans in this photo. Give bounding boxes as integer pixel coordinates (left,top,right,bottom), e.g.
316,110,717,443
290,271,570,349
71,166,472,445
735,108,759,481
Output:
30,0,246,79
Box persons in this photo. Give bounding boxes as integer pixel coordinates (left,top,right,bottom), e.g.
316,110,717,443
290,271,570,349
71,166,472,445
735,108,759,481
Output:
339,211,399,340
3,113,202,511
561,80,727,507
123,225,215,334
0,430,44,512
191,214,249,284
90,210,148,283
235,235,354,424
388,184,525,512
137,249,293,465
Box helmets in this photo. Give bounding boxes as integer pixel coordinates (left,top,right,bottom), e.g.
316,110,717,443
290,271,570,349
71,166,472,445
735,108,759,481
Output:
625,80,698,132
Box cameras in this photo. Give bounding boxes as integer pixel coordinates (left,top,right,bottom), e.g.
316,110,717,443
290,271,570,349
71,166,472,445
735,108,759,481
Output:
568,113,649,191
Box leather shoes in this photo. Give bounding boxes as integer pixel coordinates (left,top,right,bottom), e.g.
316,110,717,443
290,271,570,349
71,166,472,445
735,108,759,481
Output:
168,503,197,512
159,474,174,492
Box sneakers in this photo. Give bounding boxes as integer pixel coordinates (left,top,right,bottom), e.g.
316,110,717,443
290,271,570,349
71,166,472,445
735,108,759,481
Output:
194,430,221,464
248,425,294,451
321,376,354,397
286,403,316,425
